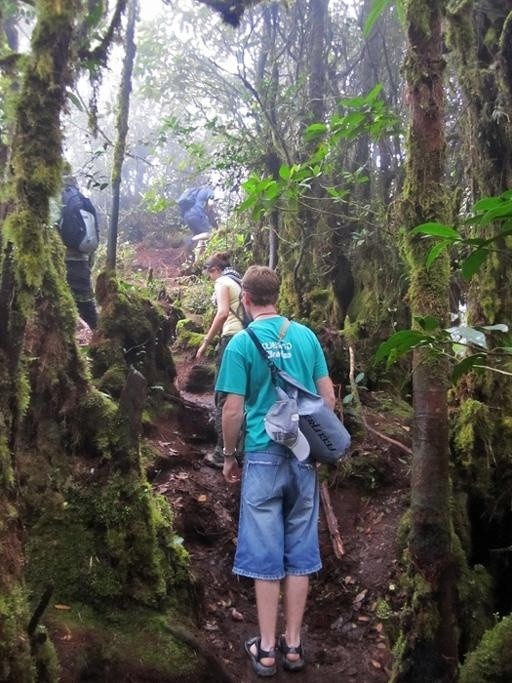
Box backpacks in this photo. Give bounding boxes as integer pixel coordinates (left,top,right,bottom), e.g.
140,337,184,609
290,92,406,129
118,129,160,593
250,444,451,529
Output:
179,187,201,210
53,186,99,253
228,275,254,329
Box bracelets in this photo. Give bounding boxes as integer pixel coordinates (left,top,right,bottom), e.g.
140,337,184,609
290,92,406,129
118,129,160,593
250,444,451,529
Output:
204,337,211,344
221,446,237,458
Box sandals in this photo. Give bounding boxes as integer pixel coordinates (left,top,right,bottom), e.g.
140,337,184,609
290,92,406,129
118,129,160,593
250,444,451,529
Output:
277,633,304,671
245,637,278,677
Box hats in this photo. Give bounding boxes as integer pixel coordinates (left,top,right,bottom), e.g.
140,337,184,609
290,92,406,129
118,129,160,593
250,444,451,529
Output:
264,400,311,461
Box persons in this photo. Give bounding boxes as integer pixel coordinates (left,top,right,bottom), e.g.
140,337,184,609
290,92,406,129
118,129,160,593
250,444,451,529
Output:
212,264,337,675
194,247,254,468
51,159,101,329
179,181,219,265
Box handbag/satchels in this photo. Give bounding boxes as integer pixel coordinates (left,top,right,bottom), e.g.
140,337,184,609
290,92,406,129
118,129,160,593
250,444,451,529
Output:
275,370,352,464
